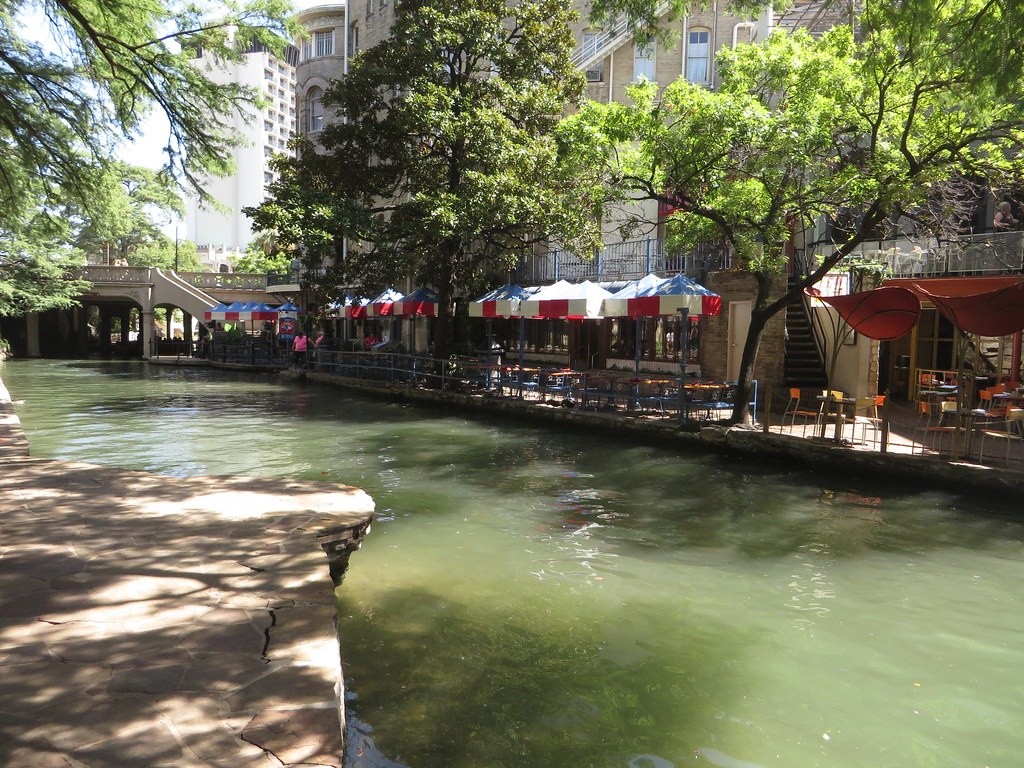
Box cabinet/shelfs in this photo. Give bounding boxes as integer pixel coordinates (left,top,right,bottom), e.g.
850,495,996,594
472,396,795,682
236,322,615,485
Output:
914,368,958,411
890,365,910,398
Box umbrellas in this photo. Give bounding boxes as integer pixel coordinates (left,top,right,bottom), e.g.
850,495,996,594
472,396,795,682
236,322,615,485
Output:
468,272,721,387
318,286,456,353
204,300,303,337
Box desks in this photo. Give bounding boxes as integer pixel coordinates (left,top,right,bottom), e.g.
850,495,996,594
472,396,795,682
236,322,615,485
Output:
511,368,538,383
642,380,670,385
682,384,729,390
548,372,581,385
814,394,857,444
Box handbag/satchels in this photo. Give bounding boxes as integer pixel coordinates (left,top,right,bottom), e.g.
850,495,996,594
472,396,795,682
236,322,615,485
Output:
313,351,316,360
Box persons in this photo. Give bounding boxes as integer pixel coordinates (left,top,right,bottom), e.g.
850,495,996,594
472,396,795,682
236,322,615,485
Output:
492,339,504,365
292,331,325,370
364,332,381,350
994,202,1022,232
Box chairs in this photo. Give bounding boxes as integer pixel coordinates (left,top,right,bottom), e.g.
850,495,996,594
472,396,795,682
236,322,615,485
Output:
935,400,976,455
780,388,817,439
500,364,758,425
817,390,847,441
975,381,1024,421
912,400,954,456
979,408,1024,464
980,405,1021,458
851,395,891,448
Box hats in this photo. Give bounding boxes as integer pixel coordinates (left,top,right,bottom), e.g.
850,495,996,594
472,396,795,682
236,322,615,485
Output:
300,332,305,335
